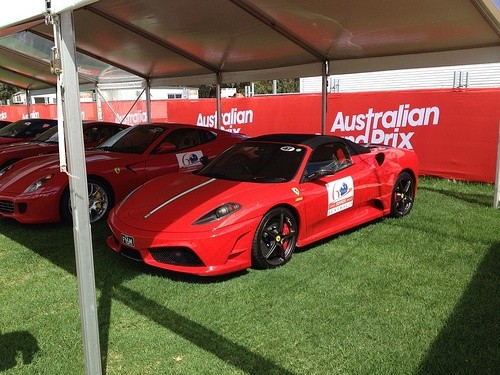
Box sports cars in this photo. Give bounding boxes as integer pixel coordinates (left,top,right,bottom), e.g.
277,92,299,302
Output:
0,116,132,170
0,118,57,144
0,122,247,231
106,133,419,277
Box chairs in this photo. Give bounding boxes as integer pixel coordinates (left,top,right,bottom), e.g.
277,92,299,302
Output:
267,147,292,178
313,147,338,176
